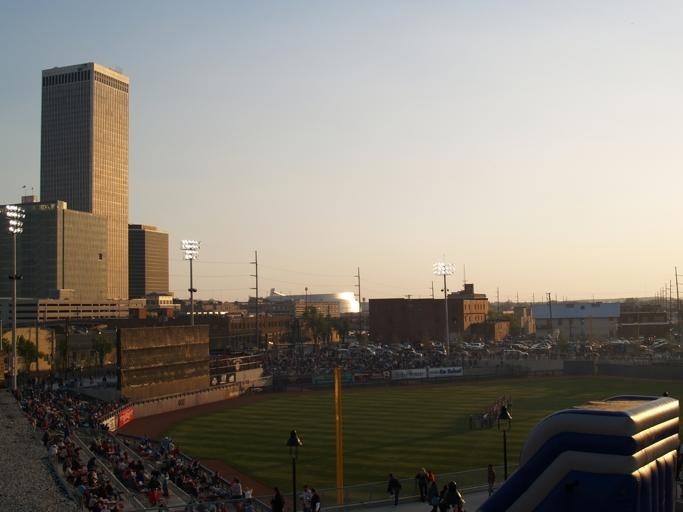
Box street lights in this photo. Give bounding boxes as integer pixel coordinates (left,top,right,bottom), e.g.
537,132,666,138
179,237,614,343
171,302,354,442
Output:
432,261,456,358
496,405,512,480
286,431,303,512
3,204,28,391
304,285,308,312
182,240,201,325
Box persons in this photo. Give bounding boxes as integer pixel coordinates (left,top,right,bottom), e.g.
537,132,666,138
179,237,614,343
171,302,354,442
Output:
417,468,463,512
300,485,321,512
269,486,285,512
7,372,256,510
488,464,496,497
386,475,402,506
213,342,511,377
471,397,510,428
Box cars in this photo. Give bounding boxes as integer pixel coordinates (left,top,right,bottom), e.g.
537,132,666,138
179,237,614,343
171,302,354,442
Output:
307,341,552,363
553,334,670,356
